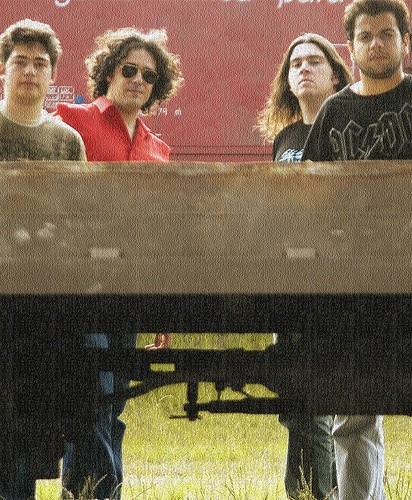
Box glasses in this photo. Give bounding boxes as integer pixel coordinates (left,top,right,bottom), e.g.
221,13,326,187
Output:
117,63,160,84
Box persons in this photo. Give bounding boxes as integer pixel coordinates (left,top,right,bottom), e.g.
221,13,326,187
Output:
50,26,182,500
3,17,87,499
300,0,412,499
258,32,354,500
